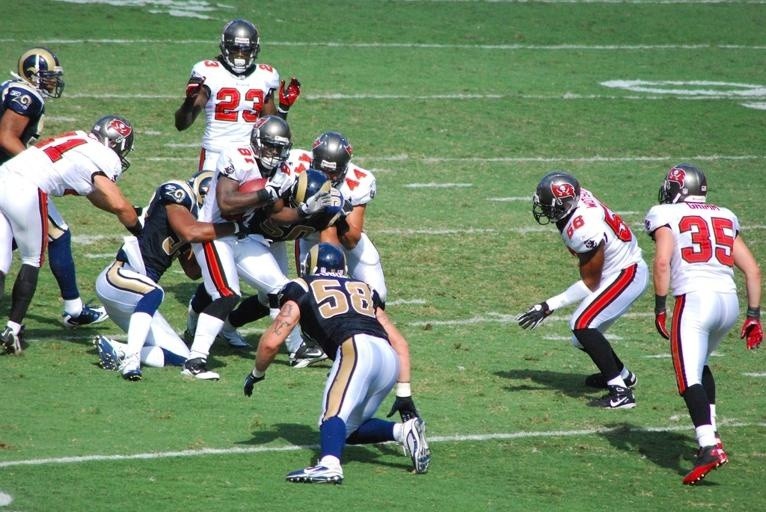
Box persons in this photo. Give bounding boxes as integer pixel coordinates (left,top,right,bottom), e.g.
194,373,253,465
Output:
515,170,650,410
184,168,353,350
243,242,432,483
286,131,388,306
0,114,142,356
180,115,330,380
644,162,763,484
92,171,259,380
174,20,301,169
0,47,111,327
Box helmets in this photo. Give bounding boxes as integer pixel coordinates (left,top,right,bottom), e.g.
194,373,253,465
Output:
312,132,351,184
17,47,64,99
92,116,134,155
291,169,331,221
187,170,214,207
303,241,347,277
219,19,263,72
533,172,579,224
658,164,707,204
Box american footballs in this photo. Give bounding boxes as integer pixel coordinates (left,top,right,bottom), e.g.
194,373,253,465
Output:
240,179,267,192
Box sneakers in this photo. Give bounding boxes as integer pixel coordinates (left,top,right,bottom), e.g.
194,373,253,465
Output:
62,304,111,329
285,459,344,485
92,335,143,381
399,416,431,474
218,327,251,347
586,387,637,409
181,357,221,380
287,341,330,368
1,325,24,357
682,430,728,486
584,370,638,389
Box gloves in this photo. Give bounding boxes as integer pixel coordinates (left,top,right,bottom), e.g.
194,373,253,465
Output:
238,208,261,234
279,74,301,114
386,393,420,422
740,318,763,351
653,308,669,341
299,192,333,218
186,71,206,100
249,115,294,169
243,369,265,397
264,162,297,203
517,299,555,331
